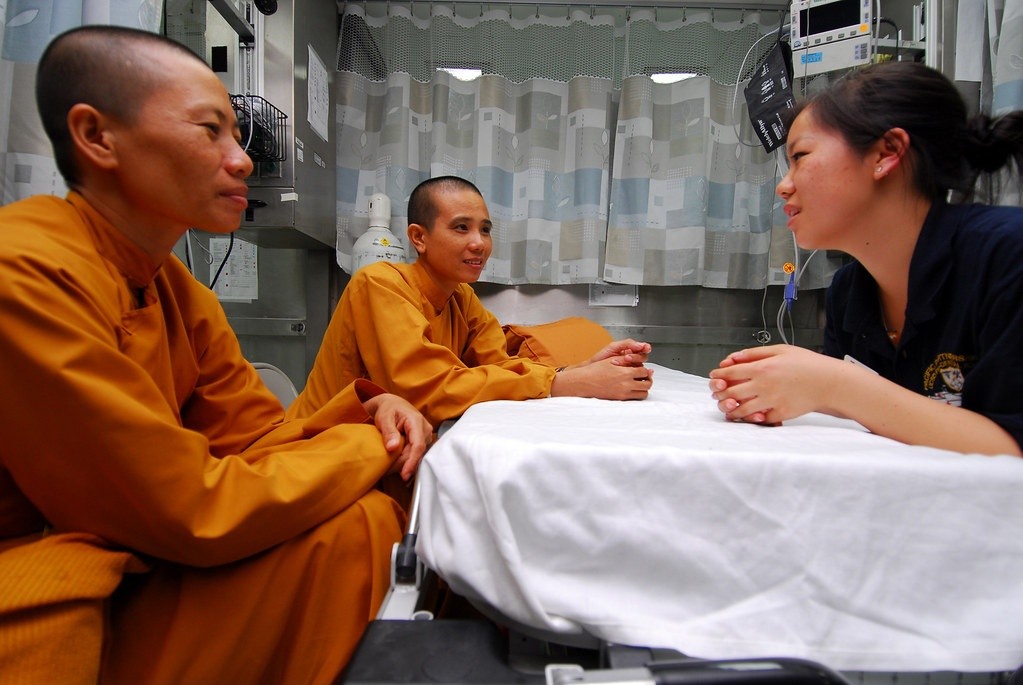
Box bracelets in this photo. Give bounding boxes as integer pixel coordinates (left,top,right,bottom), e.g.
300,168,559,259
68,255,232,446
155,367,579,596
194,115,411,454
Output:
555,367,566,372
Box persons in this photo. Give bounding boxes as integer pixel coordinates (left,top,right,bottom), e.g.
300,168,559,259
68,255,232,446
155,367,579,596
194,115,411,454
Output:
708,61,1023,455
285,176,654,501
0,23,434,685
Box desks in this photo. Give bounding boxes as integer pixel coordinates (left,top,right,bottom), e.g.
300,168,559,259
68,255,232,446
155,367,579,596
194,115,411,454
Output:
404,362,1023,685
